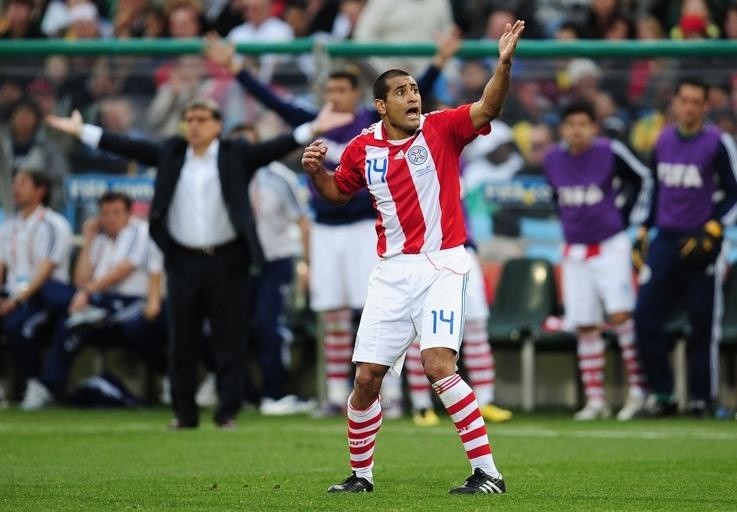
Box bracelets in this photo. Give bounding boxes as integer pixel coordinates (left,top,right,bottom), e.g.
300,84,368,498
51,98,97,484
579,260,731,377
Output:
12,293,22,308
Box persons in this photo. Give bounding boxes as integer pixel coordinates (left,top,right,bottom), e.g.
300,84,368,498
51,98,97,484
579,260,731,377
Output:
64,191,151,408
142,231,222,407
2,1,737,257
543,100,655,420
302,19,528,496
0,169,73,410
202,23,463,428
406,149,515,426
635,77,737,417
228,122,313,416
43,95,352,433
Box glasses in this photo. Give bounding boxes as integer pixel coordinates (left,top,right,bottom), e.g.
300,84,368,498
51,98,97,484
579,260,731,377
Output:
187,118,206,122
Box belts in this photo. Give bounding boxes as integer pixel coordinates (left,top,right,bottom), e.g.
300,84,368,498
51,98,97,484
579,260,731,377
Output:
170,237,242,256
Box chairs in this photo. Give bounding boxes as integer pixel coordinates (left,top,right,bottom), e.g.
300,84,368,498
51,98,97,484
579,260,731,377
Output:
484,256,554,412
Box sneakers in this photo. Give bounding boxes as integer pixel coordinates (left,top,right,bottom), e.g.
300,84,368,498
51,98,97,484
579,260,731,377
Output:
327,471,373,493
260,395,317,414
479,405,512,423
575,400,609,421
449,468,506,494
617,398,642,422
20,380,54,409
414,410,438,427
